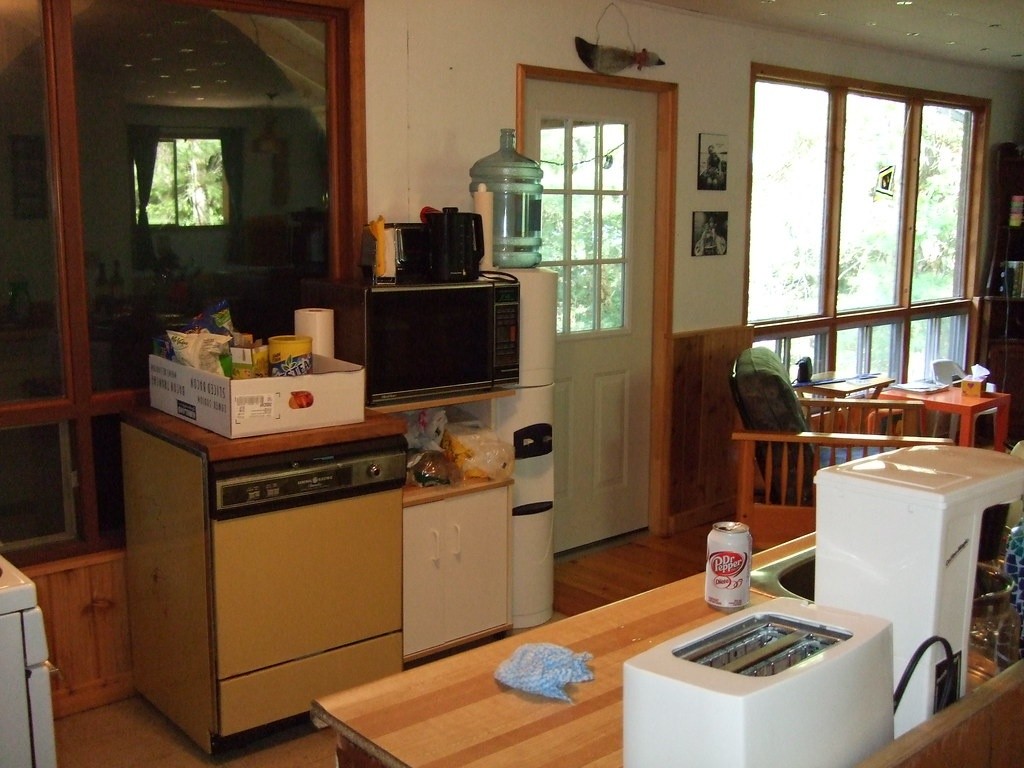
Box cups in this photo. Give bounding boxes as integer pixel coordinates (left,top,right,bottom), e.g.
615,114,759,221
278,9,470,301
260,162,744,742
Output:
996,267,1014,296
375,223,397,285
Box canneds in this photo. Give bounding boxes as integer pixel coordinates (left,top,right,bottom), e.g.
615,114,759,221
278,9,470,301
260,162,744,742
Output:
268,335,313,377
704,521,753,609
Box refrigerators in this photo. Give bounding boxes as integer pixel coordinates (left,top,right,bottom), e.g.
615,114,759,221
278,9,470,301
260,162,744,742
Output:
0,558,56,768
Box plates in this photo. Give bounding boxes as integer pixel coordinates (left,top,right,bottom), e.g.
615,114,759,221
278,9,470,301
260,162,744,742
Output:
893,379,948,394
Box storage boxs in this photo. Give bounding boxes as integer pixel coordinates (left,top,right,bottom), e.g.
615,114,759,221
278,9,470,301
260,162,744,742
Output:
148,347,366,442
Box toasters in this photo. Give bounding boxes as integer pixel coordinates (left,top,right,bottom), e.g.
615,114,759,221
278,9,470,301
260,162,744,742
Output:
623,597,894,768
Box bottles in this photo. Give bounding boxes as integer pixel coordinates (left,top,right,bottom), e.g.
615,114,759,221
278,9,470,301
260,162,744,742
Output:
470,128,542,268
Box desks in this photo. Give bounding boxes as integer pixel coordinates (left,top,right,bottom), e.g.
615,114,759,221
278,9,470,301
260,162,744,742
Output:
793,374,896,434
876,381,1012,454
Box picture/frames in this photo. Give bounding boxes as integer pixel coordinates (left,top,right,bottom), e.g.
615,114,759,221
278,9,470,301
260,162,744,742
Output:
692,211,729,256
697,133,729,190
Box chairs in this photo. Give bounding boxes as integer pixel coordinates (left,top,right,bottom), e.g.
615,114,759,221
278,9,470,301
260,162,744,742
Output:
930,359,999,449
730,395,953,551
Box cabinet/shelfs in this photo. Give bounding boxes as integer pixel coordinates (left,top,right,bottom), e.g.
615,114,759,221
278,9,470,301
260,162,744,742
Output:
371,385,515,664
975,140,1024,445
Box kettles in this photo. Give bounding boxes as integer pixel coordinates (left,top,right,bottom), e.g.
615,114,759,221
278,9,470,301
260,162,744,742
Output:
426,208,484,282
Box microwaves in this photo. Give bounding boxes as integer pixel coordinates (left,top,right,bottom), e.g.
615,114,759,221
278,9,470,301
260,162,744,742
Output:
301,279,520,407
